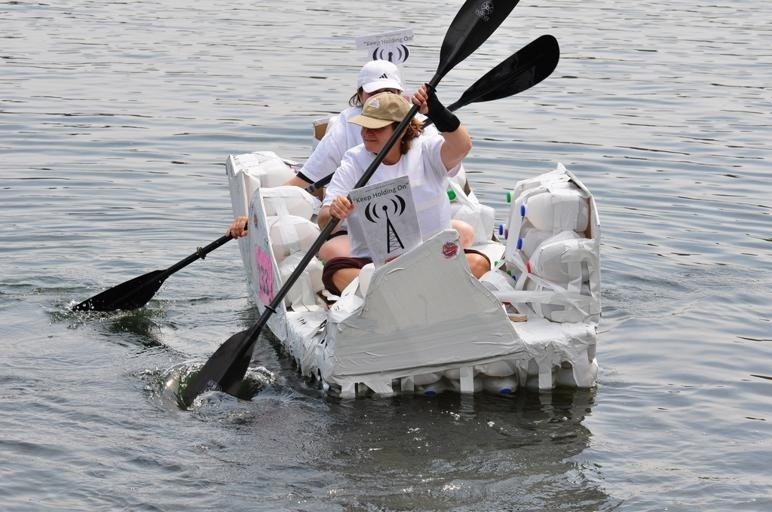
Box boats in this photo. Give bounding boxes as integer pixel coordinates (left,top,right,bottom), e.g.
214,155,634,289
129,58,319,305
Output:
226,151,600,400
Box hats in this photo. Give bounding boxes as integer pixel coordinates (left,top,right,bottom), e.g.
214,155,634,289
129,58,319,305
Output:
347,92,414,130
358,60,402,94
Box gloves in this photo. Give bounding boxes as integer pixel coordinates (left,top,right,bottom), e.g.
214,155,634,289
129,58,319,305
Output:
413,88,460,132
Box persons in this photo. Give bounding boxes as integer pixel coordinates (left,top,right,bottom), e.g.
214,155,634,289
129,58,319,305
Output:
225,58,475,260
317,83,493,294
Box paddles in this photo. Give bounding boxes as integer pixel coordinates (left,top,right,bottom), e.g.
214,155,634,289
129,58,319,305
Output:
72,34,559,314
178,1,520,407
